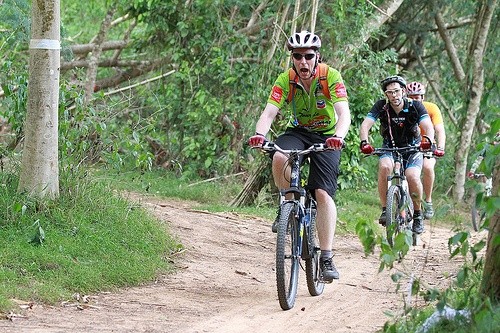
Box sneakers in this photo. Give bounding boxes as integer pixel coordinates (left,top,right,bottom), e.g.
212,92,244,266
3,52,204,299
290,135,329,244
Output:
424,204,433,220
378,212,386,224
319,256,339,280
412,215,423,234
272,210,280,232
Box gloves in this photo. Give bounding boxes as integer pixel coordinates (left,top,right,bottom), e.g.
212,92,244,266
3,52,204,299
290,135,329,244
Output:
433,150,444,156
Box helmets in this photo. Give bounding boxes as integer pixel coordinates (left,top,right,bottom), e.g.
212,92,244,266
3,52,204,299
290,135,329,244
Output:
406,82,425,94
380,75,407,91
286,30,322,51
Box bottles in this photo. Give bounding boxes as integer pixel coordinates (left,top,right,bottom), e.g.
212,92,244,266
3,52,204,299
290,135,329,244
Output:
401,176,409,195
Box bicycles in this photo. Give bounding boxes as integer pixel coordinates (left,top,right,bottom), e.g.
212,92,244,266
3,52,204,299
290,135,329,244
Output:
243,139,347,311
471,172,492,231
361,142,441,249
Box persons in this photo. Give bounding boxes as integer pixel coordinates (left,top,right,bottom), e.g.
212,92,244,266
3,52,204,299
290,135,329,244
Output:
360,75,500,234
247,30,352,279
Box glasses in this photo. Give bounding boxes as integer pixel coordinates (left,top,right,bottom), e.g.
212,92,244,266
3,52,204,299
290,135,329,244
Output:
384,90,401,94
291,53,316,60
